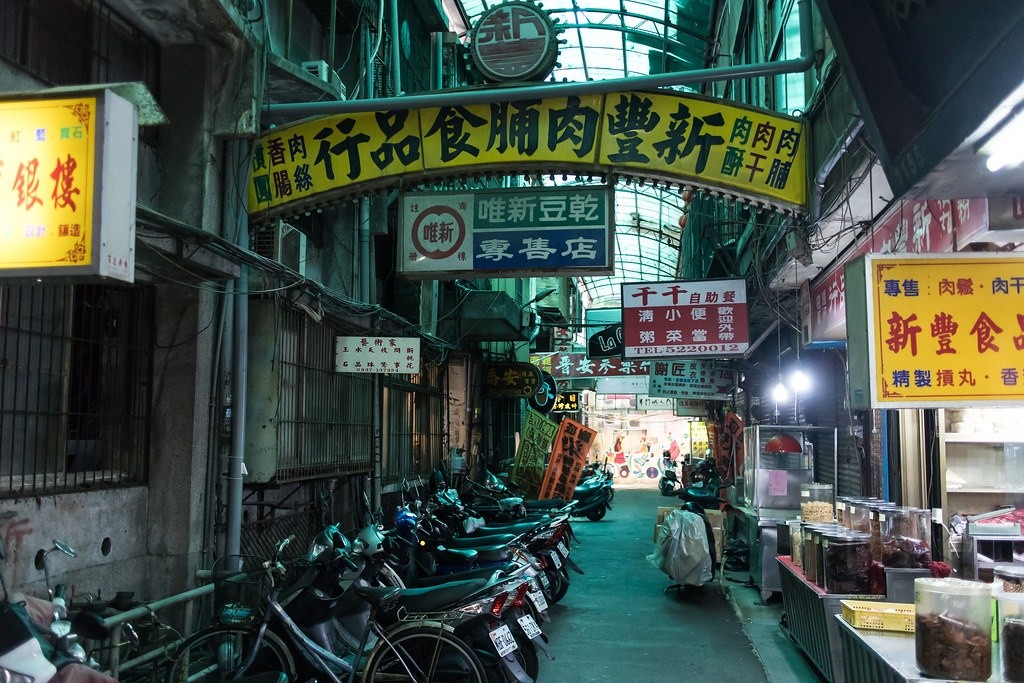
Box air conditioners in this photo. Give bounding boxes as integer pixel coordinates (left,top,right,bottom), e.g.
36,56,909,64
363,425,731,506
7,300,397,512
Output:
301,59,347,102
255,218,307,276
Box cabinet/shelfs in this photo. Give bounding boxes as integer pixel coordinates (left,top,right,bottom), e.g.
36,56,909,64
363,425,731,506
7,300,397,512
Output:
938,408,1024,577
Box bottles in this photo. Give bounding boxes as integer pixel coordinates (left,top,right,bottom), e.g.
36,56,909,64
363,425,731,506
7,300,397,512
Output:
997,593,1024,682
790,483,931,594
914,577,992,682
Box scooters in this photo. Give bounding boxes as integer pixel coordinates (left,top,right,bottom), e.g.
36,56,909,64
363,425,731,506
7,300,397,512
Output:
541,454,615,522
663,470,736,598
658,458,678,496
267,451,585,683
681,449,721,512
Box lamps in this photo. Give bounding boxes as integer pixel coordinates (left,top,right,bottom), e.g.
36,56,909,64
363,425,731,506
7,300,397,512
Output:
535,170,543,180
600,172,608,183
470,170,493,183
768,205,803,225
573,171,582,181
548,170,558,180
497,170,517,182
423,176,441,189
562,169,569,180
716,191,738,206
256,185,395,232
444,174,468,187
587,173,593,182
638,176,659,190
520,171,531,182
691,186,711,201
741,198,764,214
614,174,633,185
664,181,685,195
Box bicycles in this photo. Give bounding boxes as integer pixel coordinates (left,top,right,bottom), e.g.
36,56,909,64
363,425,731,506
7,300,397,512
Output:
162,536,489,683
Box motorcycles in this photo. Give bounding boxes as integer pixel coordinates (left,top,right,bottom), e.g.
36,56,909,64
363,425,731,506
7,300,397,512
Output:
34,541,138,683
